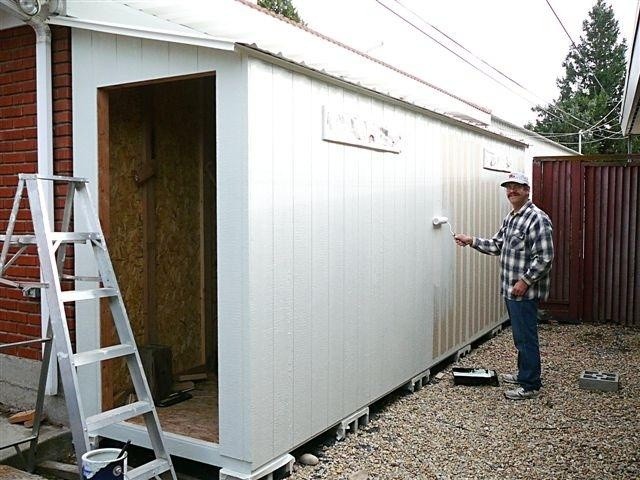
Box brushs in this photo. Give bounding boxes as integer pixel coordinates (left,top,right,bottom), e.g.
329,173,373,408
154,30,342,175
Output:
433,215,466,247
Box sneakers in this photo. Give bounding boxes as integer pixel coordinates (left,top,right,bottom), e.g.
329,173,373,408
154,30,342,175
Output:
503,385,544,400
499,373,521,384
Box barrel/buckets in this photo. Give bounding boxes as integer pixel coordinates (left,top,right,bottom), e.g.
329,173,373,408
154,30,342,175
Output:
81,448,128,480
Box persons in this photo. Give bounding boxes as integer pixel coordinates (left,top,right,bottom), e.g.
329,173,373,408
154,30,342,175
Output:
453,170,556,401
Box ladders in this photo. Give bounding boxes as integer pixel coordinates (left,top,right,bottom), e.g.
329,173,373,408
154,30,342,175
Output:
0,171,179,480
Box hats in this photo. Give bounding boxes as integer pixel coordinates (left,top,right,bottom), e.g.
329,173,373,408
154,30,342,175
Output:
501,173,530,188
